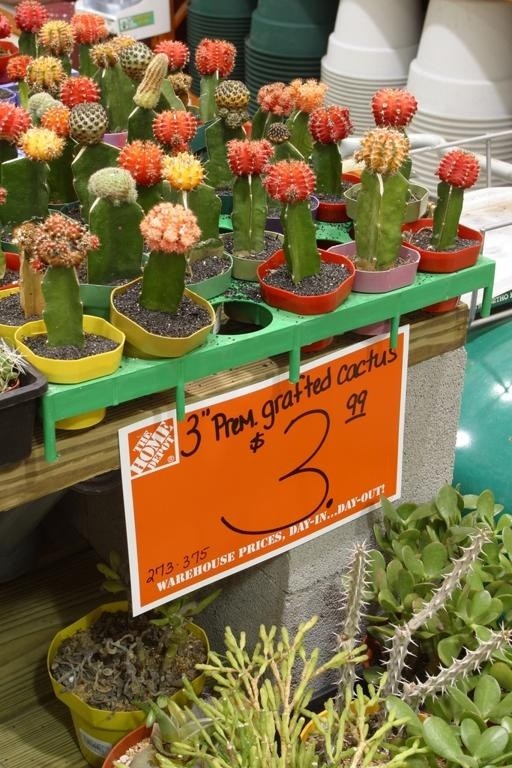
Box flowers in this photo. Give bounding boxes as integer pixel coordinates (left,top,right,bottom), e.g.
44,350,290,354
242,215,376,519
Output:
428,149,480,252
0,101,33,165
60,74,100,110
262,160,327,281
154,40,189,72
40,105,79,202
0,10,13,41
167,71,199,115
354,126,409,271
14,0,48,60
8,56,33,111
0,127,67,230
86,166,147,284
252,79,326,158
141,201,202,317
121,41,188,113
371,85,418,202
227,139,275,254
25,55,65,101
89,36,136,134
12,217,45,318
307,104,353,202
155,110,198,203
160,151,224,272
69,11,111,78
118,140,167,213
196,37,238,123
40,19,78,79
12,212,102,348
127,52,169,145
69,102,121,227
203,80,251,185
28,91,66,126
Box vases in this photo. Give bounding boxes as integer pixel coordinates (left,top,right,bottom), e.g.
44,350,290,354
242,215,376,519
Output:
246,0,328,123
320,0,423,136
8,83,20,106
256,246,355,353
186,0,250,97
213,187,233,216
49,199,80,215
0,40,19,85
105,129,127,148
319,172,362,221
80,253,151,323
184,251,233,299
342,183,432,224
12,313,127,429
266,194,319,234
407,113,511,187
112,277,218,361
405,0,512,123
219,231,285,283
399,218,486,314
0,287,43,348
329,240,419,337
1,87,16,104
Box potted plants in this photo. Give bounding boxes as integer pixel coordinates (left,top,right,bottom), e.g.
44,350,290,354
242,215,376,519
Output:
0,338,47,465
0,250,20,289
296,486,511,768
46,540,223,768
102,616,432,768
0,221,18,253
